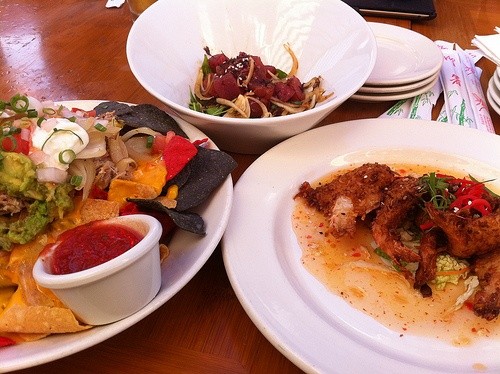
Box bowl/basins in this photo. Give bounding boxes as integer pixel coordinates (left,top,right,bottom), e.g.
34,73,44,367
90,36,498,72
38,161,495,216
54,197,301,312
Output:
32,213,163,325
126,1,377,155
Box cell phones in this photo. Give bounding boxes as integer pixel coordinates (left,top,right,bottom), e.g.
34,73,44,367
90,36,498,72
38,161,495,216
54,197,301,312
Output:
342,0,437,20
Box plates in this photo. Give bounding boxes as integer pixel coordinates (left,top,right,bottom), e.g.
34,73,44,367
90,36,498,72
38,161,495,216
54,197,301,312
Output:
487,65,500,116
348,22,444,101
1,99,233,373
222,118,499,373
